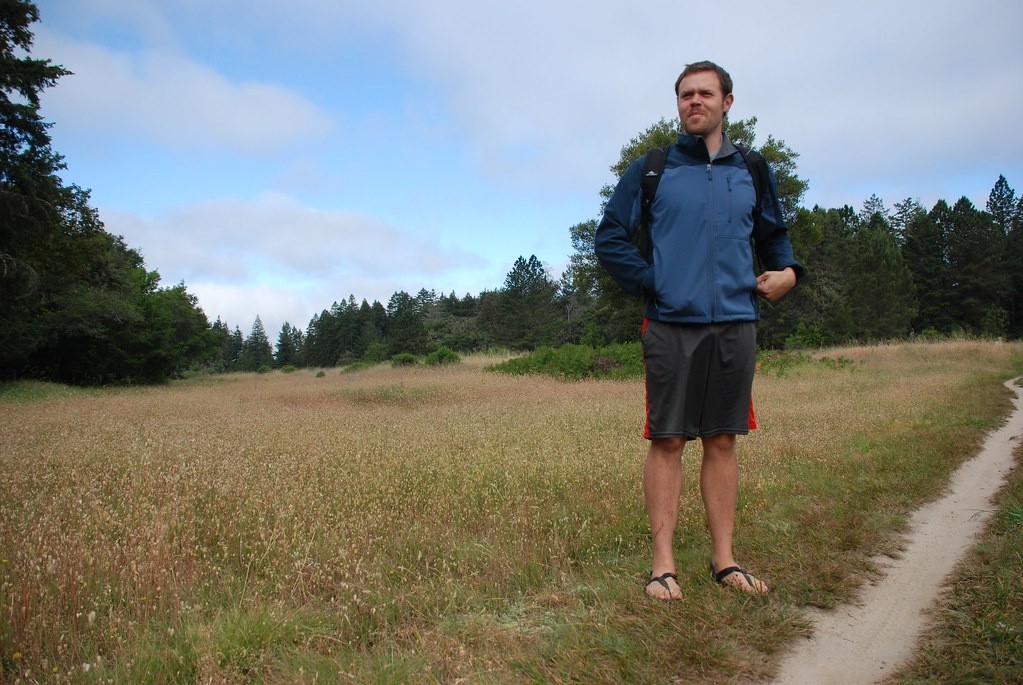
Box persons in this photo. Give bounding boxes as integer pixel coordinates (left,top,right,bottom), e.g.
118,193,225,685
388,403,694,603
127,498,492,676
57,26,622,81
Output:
593,57,808,602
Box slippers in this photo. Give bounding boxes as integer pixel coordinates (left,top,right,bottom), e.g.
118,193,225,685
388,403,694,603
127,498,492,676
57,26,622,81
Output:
714,566,771,597
646,570,683,604
